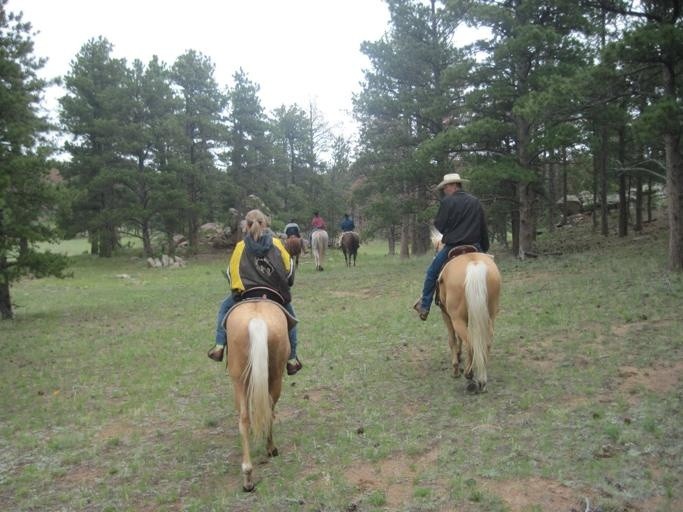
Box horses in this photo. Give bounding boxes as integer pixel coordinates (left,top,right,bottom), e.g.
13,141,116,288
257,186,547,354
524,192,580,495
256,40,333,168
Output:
283,234,303,271
340,231,359,268
428,227,503,395
220,268,294,493
310,229,329,271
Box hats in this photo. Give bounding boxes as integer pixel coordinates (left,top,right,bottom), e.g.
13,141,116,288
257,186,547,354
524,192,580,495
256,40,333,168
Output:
436,173,470,190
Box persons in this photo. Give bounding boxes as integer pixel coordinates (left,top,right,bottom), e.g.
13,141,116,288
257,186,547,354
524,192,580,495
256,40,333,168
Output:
414,173,490,320
208,209,302,375
312,210,324,229
339,213,355,247
284,217,309,253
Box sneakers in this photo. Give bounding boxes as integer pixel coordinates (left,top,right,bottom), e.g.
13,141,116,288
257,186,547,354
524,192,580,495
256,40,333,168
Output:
208,347,224,361
413,297,430,320
287,357,302,375
